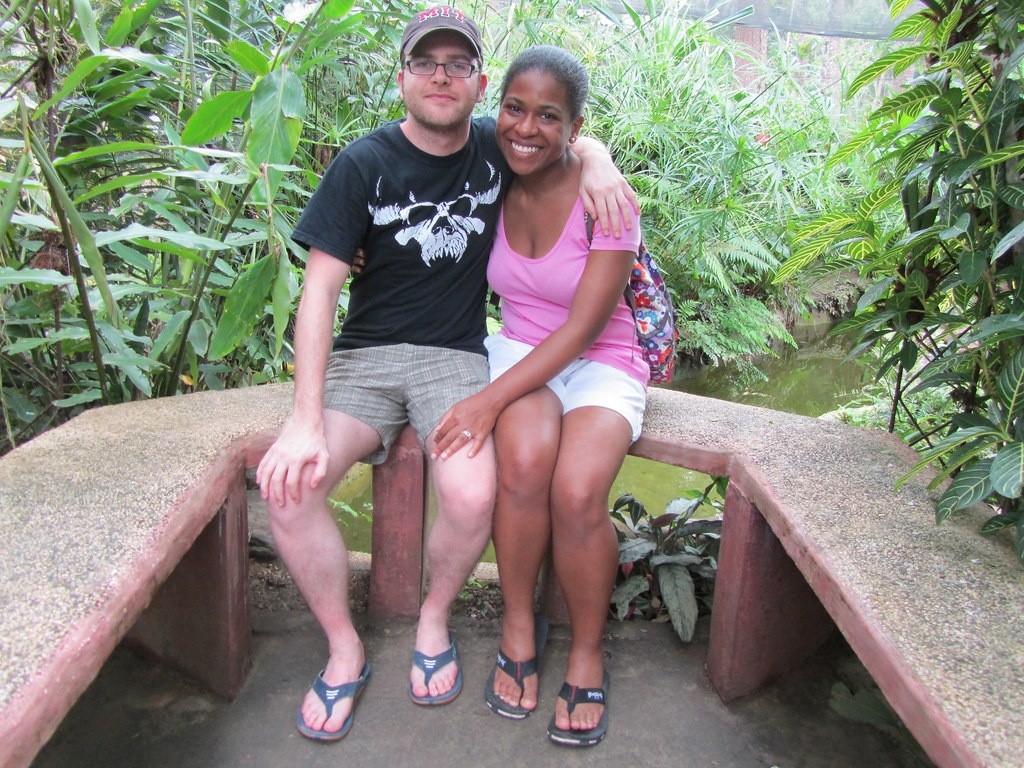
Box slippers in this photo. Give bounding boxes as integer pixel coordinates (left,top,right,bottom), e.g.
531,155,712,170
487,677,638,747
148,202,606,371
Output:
483,613,550,720
545,668,610,748
295,660,371,742
408,629,464,706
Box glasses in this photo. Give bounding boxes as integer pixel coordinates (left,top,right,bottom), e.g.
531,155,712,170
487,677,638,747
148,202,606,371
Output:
401,60,482,78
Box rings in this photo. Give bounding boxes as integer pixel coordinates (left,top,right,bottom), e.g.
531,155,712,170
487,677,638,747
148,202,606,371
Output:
462,430,471,440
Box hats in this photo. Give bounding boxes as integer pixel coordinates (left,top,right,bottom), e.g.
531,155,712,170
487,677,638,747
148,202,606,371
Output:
399,4,484,68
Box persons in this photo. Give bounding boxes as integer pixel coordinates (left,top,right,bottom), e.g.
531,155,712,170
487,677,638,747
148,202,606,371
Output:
352,44,650,746
253,7,642,741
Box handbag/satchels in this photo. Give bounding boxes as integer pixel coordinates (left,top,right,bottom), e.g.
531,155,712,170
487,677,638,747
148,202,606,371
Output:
582,210,680,384
